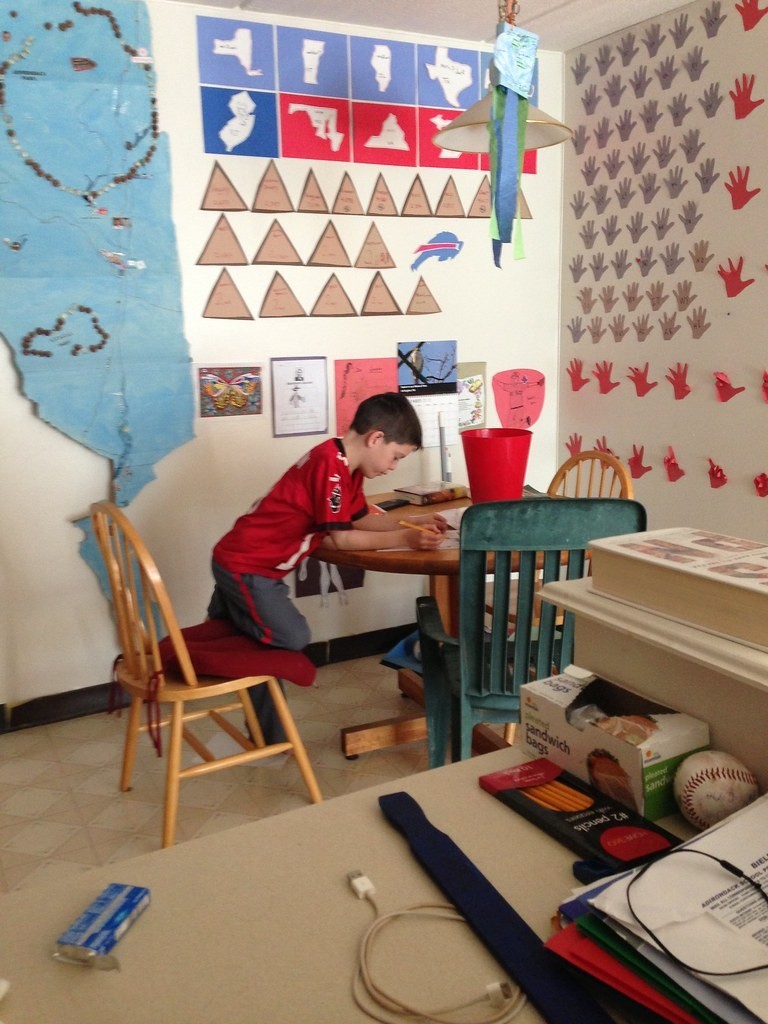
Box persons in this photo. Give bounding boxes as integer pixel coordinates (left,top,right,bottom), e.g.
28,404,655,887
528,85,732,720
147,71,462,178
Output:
205,392,449,757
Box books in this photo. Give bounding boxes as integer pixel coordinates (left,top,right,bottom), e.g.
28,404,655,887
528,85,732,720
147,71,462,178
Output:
394,479,467,506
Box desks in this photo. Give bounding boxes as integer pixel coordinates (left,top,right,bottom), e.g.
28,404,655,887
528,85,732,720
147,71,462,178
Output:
307,487,591,762
0,742,768,1024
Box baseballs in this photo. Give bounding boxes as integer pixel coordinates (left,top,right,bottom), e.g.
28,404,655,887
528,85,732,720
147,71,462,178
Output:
674,749,759,831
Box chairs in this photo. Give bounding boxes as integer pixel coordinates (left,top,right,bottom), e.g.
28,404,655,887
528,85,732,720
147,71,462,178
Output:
484,450,633,638
89,500,323,850
417,496,648,768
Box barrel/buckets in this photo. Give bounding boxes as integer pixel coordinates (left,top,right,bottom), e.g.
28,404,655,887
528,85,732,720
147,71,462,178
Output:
461,428,534,505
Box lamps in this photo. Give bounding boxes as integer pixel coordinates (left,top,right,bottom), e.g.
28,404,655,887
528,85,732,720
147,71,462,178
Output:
430,0,574,270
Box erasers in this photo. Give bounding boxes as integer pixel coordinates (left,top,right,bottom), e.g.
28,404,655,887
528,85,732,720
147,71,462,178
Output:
53,883,151,966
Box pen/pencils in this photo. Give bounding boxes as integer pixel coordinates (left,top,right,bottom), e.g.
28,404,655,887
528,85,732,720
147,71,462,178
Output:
399,520,450,539
518,780,594,811
